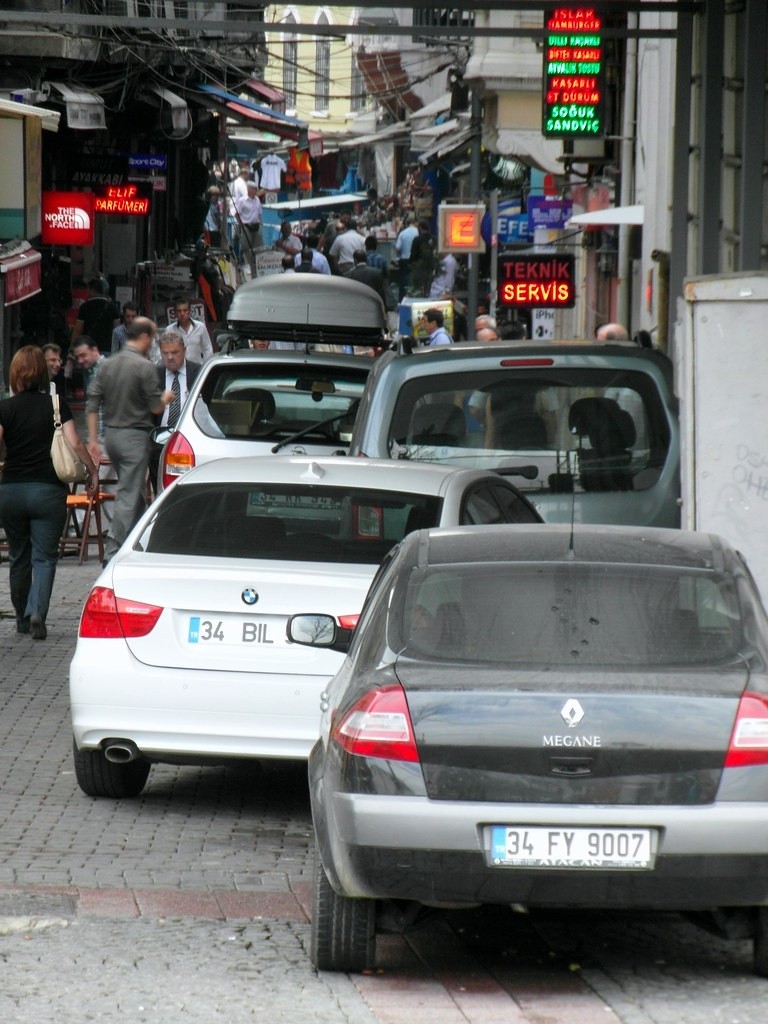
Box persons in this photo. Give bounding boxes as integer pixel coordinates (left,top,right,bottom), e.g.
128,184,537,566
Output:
597,322,628,340
85,316,177,568
41,267,213,571
272,188,461,312
0,343,99,644
453,314,547,451
201,156,263,273
422,309,455,403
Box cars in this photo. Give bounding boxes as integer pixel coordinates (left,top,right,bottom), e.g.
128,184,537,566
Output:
358,337,686,530
69,457,548,797
284,525,768,973
157,349,381,503
224,272,388,360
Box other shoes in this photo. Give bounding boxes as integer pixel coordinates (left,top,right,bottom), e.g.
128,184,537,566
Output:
30,616,47,640
17,624,28,633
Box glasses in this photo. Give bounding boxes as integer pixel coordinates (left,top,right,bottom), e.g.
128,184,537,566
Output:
46,359,64,365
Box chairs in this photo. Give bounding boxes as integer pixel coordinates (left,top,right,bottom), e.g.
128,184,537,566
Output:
58,477,118,567
224,386,278,438
568,397,620,452
220,517,284,558
413,404,467,449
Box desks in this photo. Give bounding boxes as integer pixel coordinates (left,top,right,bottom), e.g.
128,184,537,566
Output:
60,459,115,559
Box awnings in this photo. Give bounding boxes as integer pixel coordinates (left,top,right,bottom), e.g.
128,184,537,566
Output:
564,202,644,229
408,116,461,152
0,237,43,306
256,123,325,158
243,75,285,104
336,129,394,147
187,80,310,149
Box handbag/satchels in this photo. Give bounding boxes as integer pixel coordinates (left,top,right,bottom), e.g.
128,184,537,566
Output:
48,382,87,483
244,223,260,233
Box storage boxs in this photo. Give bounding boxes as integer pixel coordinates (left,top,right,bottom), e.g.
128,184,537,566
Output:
210,400,262,435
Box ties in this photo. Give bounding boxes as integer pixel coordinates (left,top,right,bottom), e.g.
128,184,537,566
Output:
168,371,181,427
